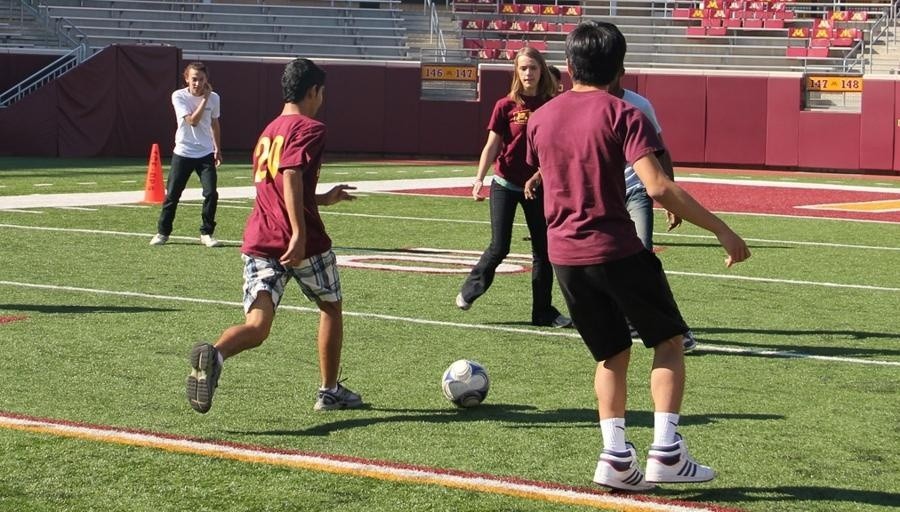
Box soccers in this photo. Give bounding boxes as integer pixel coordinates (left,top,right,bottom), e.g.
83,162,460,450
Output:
440,360,490,408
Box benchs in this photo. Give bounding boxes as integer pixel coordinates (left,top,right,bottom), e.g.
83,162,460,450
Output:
50,0,416,56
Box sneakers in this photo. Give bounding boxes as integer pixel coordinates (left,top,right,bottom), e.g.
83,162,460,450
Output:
645,434,716,482
150,234,168,246
549,315,572,329
593,449,657,492
314,385,362,412
187,342,223,412
200,233,221,247
456,291,471,311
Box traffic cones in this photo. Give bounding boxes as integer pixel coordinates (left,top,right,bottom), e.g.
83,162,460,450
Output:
133,144,169,207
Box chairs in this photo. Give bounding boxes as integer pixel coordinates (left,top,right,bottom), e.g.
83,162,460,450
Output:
668,0,803,40
456,1,585,63
782,9,874,61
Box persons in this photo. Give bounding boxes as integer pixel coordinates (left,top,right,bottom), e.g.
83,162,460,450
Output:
526,19,752,491
149,62,223,248
523,63,697,352
185,58,363,413
455,47,571,330
546,65,561,88
